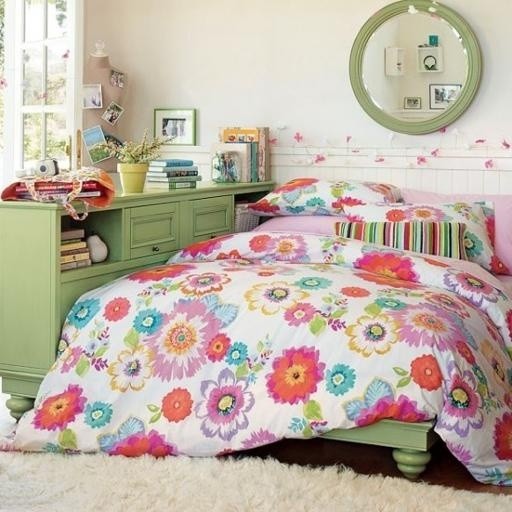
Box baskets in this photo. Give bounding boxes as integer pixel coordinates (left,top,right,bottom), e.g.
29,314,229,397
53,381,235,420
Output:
233,205,261,233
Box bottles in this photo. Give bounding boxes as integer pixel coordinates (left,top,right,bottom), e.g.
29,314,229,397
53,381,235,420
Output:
210,151,243,184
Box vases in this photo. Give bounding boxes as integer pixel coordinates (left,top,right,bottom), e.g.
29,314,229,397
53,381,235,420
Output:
115,159,149,194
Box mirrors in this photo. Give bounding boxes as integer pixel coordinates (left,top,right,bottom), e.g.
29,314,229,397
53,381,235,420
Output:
349,0,484,137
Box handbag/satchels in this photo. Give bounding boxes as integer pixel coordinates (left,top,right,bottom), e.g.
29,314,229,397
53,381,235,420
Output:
1,165,117,223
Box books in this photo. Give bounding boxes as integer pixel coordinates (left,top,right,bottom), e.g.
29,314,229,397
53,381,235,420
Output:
144,158,201,189
59,229,91,270
209,126,271,183
16,182,100,202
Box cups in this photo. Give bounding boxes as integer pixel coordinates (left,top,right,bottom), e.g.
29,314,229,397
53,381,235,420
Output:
429,33,439,46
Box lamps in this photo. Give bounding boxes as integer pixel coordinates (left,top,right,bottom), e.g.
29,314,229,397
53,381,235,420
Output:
90,43,132,147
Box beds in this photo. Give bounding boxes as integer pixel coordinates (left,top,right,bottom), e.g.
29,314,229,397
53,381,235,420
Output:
1,230,512,489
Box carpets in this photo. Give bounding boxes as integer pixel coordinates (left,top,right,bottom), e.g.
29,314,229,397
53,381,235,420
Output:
1,441,511,511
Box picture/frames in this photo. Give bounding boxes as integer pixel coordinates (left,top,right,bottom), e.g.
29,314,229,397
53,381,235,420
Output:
385,45,408,76
403,98,420,109
427,82,463,113
81,83,103,111
417,46,443,74
100,102,126,127
152,107,198,148
83,125,110,163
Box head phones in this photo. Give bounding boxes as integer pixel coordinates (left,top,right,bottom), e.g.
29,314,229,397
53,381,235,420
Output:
16,158,58,178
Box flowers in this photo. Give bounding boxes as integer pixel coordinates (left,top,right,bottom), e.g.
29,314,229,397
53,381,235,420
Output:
97,127,178,164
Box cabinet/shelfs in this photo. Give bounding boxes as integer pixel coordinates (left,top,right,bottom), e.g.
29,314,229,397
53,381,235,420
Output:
1,181,276,420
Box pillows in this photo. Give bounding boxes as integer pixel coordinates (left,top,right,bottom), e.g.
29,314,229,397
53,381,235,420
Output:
342,200,511,279
246,177,403,219
332,216,468,263
252,214,346,241
396,186,511,269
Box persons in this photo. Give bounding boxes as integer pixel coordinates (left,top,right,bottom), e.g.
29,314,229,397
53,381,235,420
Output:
104,108,118,122
435,88,456,104
